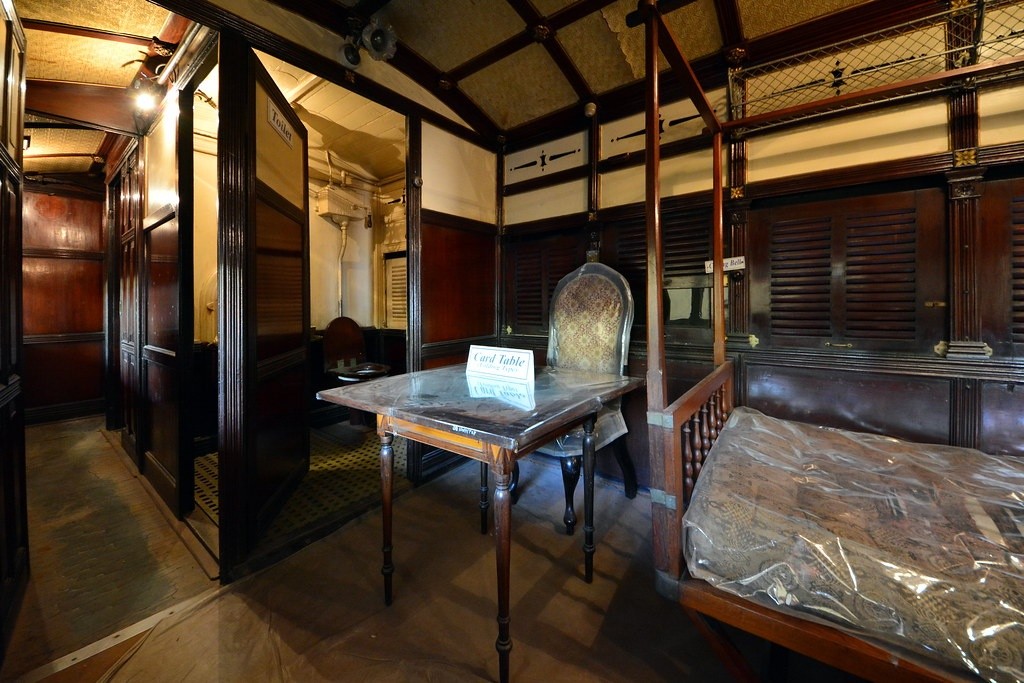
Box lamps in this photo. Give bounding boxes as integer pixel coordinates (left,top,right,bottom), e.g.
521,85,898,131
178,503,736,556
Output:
340,13,397,69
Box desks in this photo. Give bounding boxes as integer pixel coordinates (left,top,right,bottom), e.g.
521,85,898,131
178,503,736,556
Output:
317,361,646,683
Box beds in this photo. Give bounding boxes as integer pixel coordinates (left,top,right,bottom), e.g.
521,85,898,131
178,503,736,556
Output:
664,362,1024,683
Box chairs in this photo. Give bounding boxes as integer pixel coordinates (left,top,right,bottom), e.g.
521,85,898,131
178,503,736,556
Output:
505,261,639,535
